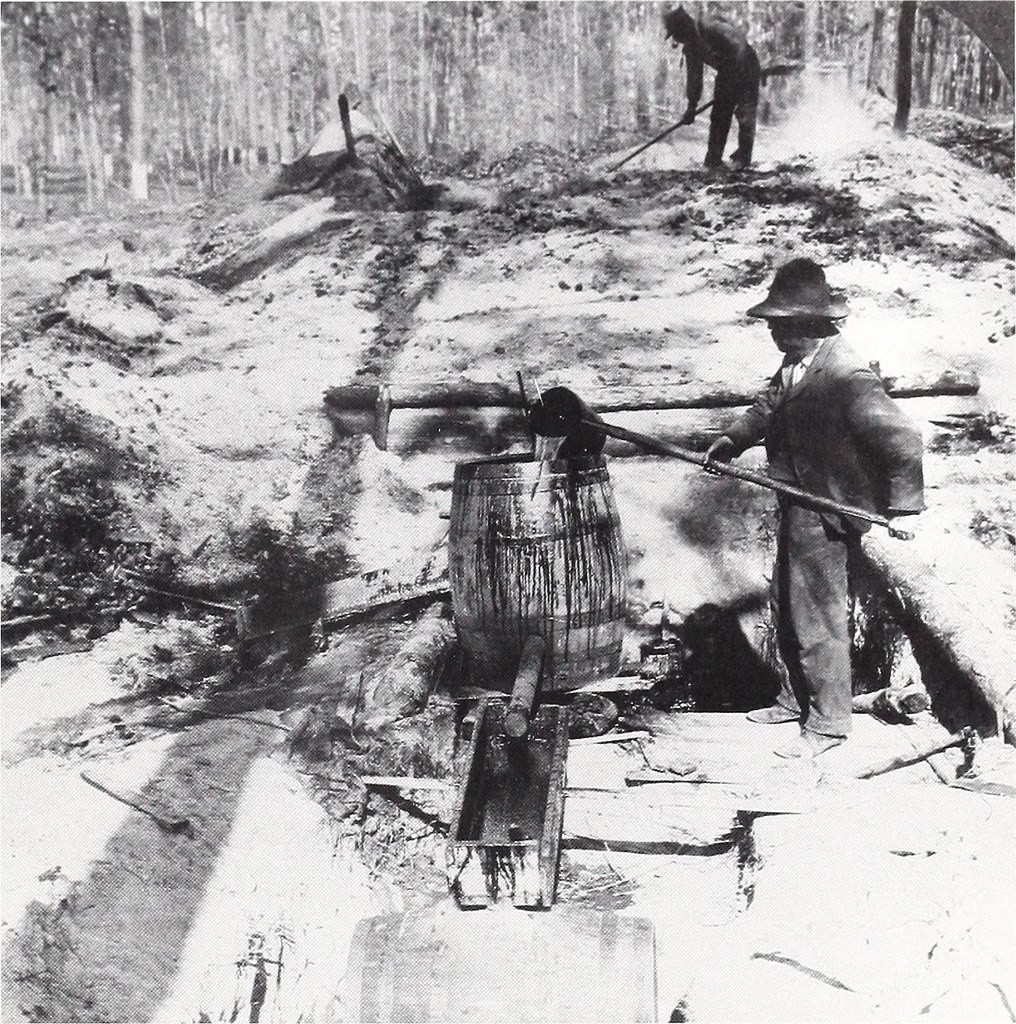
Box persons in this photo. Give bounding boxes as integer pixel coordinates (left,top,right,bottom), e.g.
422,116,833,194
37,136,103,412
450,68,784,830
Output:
663,3,761,173
702,258,928,759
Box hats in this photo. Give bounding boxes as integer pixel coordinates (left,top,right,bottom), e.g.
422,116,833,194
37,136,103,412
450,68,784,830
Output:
664,5,692,41
745,257,849,320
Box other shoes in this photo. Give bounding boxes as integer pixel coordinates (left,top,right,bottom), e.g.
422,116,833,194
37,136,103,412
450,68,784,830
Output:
774,731,841,758
731,160,744,173
745,702,804,722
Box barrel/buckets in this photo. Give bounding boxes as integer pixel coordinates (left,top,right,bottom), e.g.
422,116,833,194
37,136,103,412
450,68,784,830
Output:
449,451,624,691
